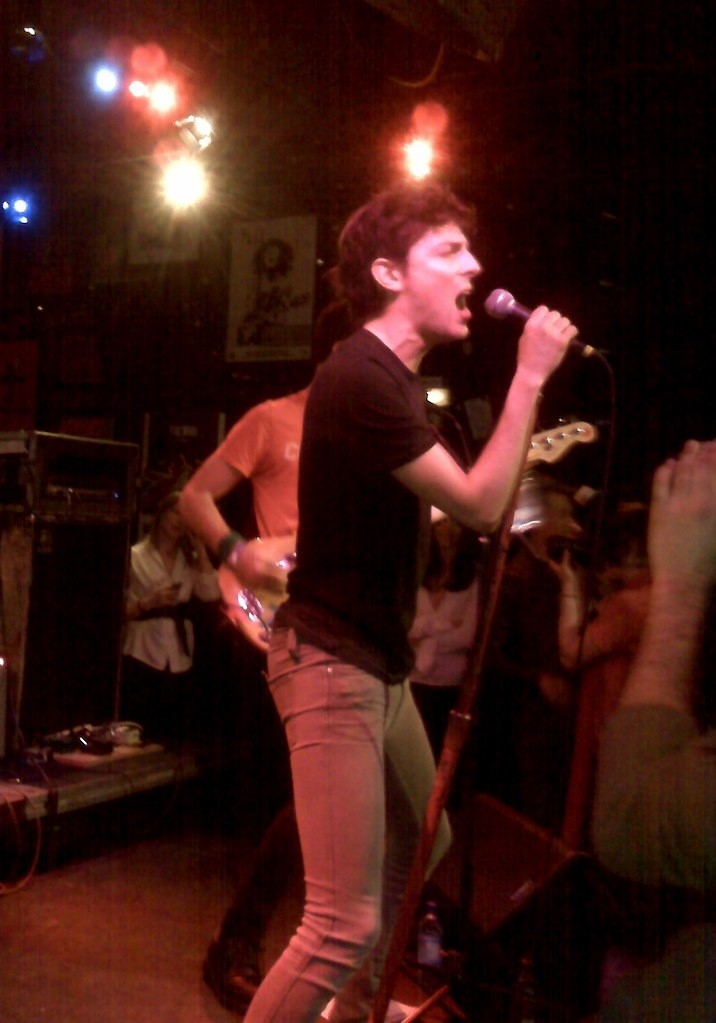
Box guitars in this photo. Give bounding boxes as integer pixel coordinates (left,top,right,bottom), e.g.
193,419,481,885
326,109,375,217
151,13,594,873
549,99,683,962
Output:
214,419,599,655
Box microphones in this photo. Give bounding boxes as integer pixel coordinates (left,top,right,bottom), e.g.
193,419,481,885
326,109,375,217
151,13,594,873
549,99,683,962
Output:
485,289,597,360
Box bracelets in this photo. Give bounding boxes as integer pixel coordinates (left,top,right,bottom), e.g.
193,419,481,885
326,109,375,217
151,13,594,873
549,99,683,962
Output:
216,532,240,559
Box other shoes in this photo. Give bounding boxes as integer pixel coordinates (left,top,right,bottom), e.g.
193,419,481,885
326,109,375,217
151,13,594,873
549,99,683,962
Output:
203,956,265,1018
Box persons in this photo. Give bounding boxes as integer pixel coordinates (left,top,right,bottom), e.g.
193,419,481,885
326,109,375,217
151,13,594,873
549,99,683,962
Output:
510,456,648,862
178,298,366,1012
408,514,483,793
243,187,578,1022
121,489,221,825
589,439,716,1022
